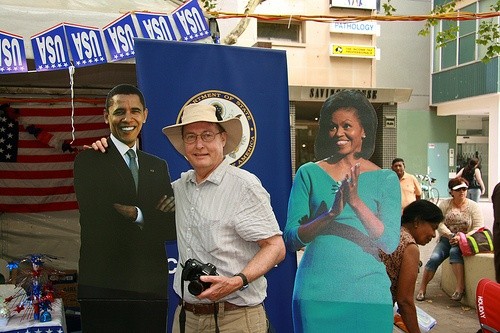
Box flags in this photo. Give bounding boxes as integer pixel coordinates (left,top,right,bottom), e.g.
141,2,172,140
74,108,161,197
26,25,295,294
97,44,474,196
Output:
0,97,112,215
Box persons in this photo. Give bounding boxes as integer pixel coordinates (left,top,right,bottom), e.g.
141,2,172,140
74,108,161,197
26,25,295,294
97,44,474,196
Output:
83,102,286,333
391,159,422,214
382,200,444,333
454,157,485,202
283,90,401,333
415,177,482,302
73,84,177,333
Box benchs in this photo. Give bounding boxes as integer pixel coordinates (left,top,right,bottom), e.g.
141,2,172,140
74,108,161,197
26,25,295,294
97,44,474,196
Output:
440,249,495,310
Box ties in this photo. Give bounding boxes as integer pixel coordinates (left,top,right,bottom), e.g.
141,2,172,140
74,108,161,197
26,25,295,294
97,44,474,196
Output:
126,149,139,195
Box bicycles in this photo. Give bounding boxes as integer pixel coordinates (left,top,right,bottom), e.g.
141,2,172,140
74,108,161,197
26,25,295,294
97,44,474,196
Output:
415,174,440,205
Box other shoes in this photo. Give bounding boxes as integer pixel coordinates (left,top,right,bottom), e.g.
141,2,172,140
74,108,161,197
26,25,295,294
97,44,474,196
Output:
451,291,463,301
416,292,425,301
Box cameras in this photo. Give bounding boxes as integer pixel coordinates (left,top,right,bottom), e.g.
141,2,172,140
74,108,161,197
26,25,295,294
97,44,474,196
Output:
183,258,220,295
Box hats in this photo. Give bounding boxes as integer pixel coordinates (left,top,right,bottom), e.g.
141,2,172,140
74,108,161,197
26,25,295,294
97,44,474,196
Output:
162,103,242,155
452,183,468,190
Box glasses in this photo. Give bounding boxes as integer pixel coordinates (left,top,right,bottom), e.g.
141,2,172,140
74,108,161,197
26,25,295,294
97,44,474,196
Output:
452,187,466,192
181,131,221,144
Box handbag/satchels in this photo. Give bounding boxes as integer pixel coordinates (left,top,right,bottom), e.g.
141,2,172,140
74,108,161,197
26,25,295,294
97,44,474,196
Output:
458,226,494,256
470,167,481,188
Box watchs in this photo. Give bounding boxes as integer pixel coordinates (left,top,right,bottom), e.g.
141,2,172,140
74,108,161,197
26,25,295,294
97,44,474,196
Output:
235,273,248,291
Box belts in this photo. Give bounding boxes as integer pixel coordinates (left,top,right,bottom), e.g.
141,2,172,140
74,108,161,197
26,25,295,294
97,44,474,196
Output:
178,297,239,315
